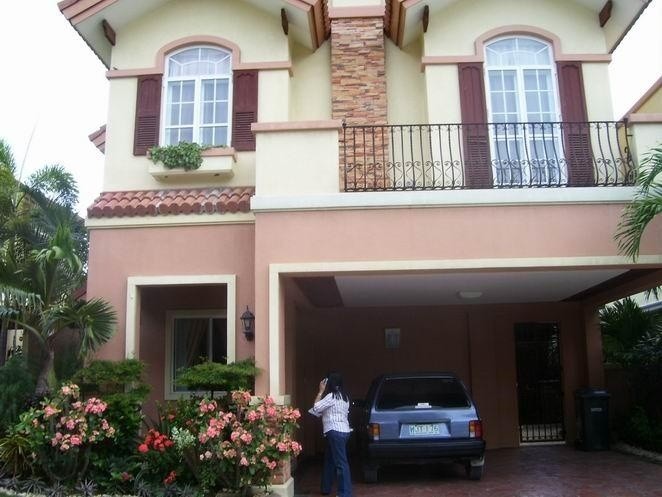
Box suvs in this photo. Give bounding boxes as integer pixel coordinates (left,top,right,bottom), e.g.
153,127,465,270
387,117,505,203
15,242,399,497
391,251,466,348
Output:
362,372,486,483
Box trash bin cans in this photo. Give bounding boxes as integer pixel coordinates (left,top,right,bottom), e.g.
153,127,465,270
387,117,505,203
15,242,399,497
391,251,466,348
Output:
574,385,612,452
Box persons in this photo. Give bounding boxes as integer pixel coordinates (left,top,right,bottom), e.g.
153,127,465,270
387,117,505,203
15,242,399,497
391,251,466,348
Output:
307,373,352,497
